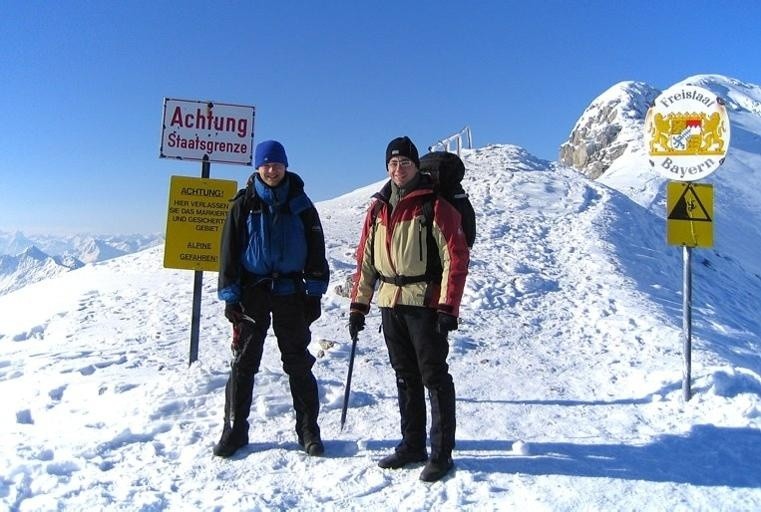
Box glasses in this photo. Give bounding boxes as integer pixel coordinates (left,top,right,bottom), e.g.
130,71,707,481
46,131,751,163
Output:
387,161,416,169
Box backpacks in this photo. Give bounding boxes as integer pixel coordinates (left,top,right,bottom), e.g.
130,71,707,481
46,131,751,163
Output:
372,151,476,249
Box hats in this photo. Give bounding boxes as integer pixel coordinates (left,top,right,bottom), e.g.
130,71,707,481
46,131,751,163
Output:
255,140,288,169
386,136,420,171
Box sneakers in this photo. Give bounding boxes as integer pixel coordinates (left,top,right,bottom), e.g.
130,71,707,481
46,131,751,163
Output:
298,433,323,457
378,448,428,469
419,454,452,480
214,421,249,456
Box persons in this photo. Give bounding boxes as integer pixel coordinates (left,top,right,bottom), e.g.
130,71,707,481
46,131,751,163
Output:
346,133,469,483
210,139,329,459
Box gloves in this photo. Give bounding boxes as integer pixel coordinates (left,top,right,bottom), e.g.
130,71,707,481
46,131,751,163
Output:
225,302,244,325
304,297,321,326
437,314,457,333
349,312,366,340
